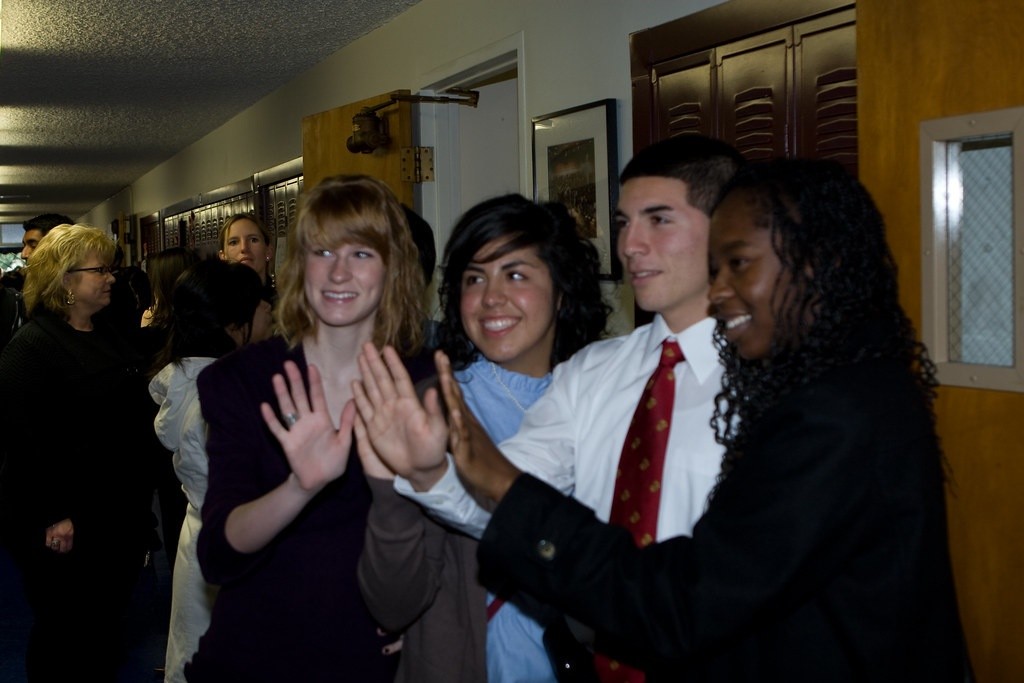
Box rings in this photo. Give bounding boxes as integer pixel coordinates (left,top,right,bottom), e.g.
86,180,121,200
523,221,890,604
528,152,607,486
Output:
52,541,58,546
285,414,298,424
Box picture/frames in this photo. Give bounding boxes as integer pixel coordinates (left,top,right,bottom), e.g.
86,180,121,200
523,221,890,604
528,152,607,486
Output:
531,98,623,281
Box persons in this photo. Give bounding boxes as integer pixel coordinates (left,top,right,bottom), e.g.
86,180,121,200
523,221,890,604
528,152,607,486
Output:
217,214,277,290
434,152,982,683
150,261,273,683
183,173,433,683
350,132,745,683
354,193,613,683
0,212,202,683
397,203,442,348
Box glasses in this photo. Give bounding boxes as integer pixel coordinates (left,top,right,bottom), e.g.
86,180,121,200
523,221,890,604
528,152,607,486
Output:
68,266,110,274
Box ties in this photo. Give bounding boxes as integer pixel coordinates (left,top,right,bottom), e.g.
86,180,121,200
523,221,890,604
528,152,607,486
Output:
593,339,686,683
